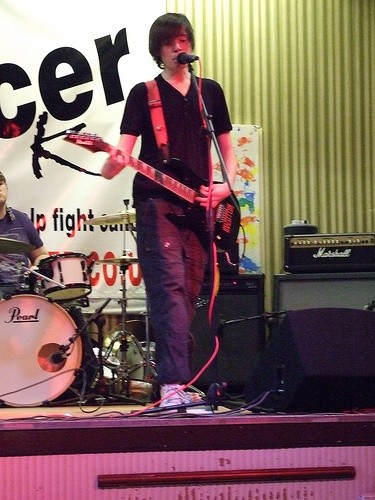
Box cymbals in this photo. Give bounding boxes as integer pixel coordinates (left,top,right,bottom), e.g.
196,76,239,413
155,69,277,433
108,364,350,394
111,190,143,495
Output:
82,207,137,225
100,256,143,263
0,237,36,255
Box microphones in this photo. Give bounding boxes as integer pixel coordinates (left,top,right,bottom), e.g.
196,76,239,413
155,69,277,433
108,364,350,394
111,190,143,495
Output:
177,52,200,64
50,352,71,363
218,315,225,335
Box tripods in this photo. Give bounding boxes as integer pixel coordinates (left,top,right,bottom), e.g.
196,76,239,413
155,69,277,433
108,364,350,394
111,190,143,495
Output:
141,310,291,415
47,261,157,407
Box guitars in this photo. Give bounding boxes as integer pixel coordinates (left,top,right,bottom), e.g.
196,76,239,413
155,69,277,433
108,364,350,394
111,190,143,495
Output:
62,133,240,251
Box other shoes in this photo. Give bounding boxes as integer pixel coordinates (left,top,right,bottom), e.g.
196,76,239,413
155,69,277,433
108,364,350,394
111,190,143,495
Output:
159,383,193,407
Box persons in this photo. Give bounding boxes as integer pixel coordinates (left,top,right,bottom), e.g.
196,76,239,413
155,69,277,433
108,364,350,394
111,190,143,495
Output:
101,13,238,408
0,171,50,299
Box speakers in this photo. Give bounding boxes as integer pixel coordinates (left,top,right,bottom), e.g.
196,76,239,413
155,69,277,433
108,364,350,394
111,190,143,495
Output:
192,271,375,411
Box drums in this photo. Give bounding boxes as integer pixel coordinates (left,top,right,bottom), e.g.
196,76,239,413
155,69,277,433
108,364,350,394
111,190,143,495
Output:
0,294,91,407
38,253,92,301
